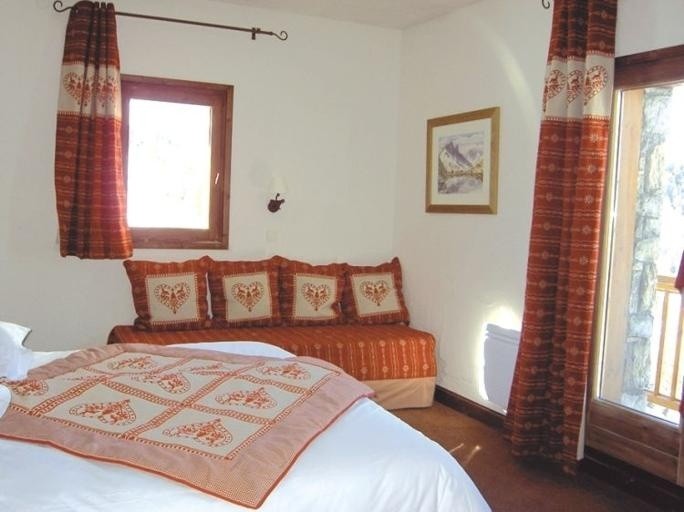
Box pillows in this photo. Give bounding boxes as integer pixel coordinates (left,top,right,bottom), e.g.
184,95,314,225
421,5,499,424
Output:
123,257,214,331
205,256,285,329
0,385,11,419
0,321,33,379
341,256,410,325
273,255,348,325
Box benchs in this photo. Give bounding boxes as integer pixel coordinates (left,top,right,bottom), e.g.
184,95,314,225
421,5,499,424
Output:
107,320,437,410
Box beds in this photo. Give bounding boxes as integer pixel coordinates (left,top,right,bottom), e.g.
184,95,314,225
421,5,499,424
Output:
0,340,493,512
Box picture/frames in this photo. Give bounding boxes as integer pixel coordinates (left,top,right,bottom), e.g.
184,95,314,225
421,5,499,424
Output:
425,106,501,214
